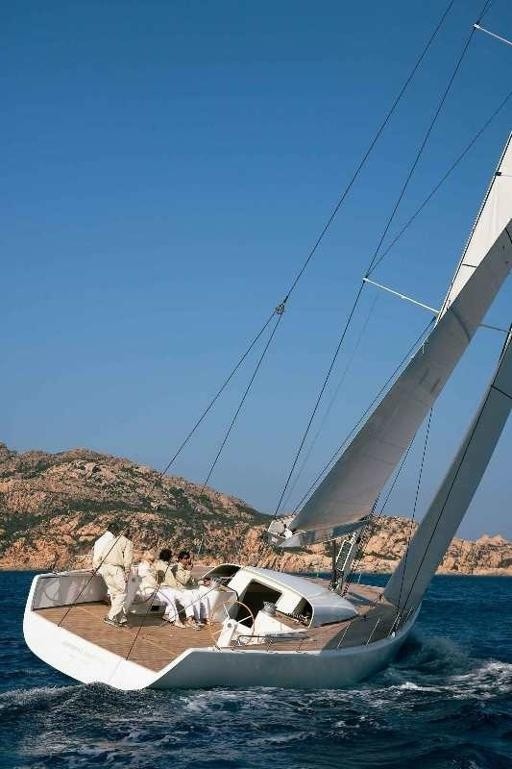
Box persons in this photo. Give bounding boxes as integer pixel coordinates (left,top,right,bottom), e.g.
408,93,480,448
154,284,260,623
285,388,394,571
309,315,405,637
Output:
153,548,200,631
100,530,135,627
92,523,121,605
171,550,216,627
138,550,188,629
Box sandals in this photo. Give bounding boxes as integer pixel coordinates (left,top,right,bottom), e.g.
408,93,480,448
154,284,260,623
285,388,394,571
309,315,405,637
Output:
162,613,216,629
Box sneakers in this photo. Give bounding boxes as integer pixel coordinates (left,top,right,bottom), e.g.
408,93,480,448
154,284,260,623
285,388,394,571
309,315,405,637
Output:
105,617,131,628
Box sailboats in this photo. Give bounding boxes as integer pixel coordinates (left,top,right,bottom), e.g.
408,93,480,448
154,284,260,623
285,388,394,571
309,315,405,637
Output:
21,3,511,689
